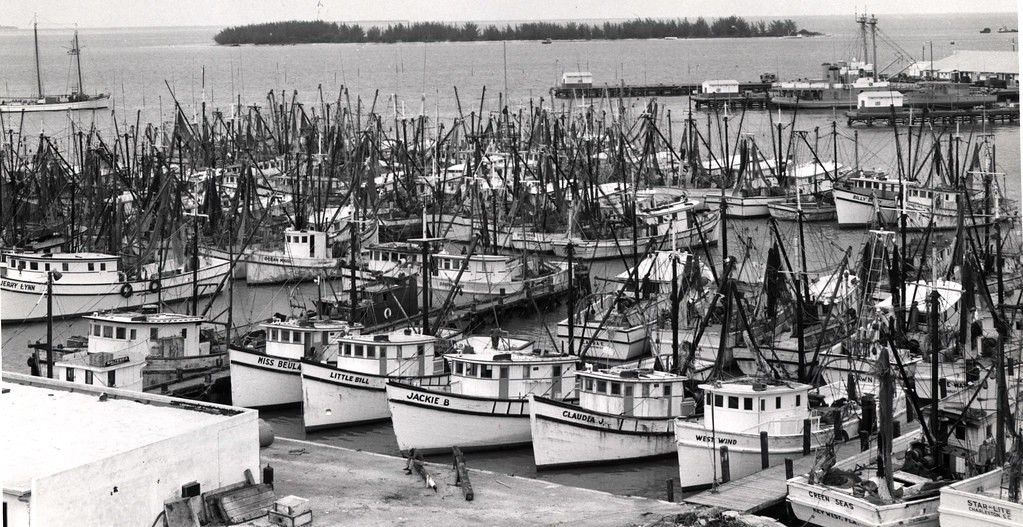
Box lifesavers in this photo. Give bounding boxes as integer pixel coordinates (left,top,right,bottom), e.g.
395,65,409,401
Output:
120,283,134,298
384,308,392,320
149,279,161,293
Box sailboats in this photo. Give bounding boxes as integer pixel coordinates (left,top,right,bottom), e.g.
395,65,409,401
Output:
0,42,1023,527
0,12,111,112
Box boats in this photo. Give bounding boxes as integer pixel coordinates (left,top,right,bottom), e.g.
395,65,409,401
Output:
784,29,803,39
771,4,998,107
541,38,552,44
997,25,1018,33
980,28,991,33
